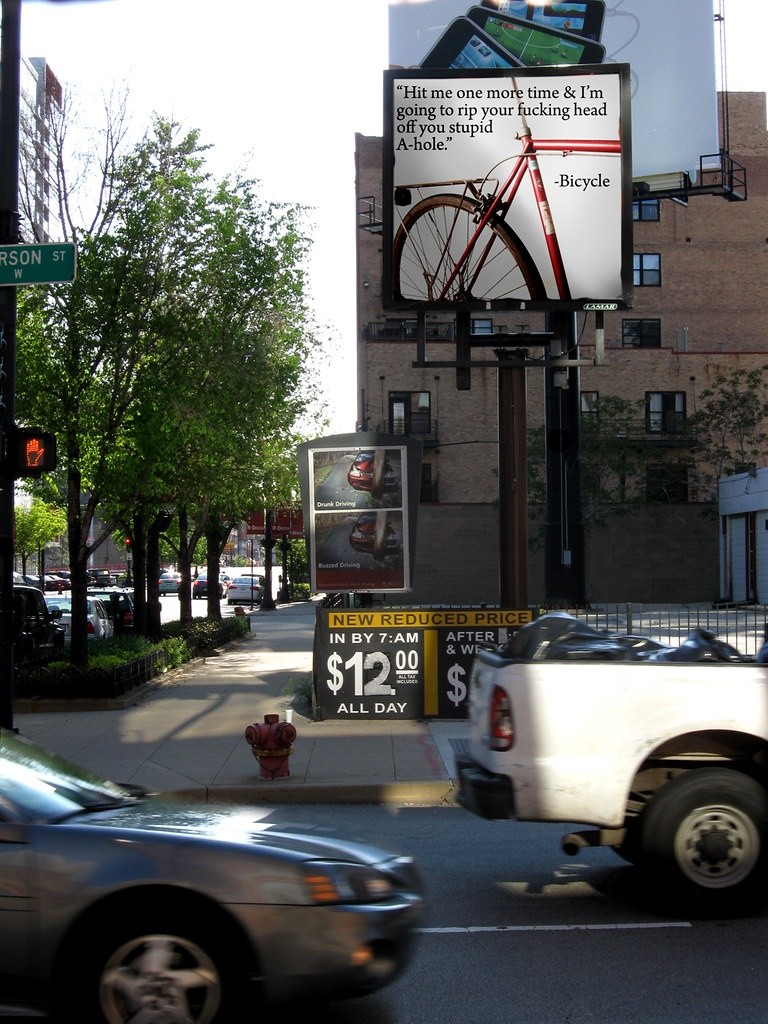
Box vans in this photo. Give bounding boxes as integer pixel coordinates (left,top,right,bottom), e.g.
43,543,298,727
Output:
90,568,111,587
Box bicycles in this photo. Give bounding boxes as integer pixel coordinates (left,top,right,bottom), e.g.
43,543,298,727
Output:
394,78,622,298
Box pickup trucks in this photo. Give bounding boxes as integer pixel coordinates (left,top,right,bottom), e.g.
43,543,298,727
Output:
446,647,768,919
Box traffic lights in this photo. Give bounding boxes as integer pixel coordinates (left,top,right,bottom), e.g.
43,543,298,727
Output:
125,538,132,552
4,426,57,481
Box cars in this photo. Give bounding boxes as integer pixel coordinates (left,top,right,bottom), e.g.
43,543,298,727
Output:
159,573,182,597
219,573,234,590
43,595,115,649
349,513,400,553
87,587,162,635
13,571,71,590
347,453,398,493
0,727,427,1024
228,576,263,605
85,570,96,586
242,574,265,596
110,576,118,586
192,572,228,600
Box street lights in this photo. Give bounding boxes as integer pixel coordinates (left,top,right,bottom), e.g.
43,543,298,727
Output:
248,539,255,611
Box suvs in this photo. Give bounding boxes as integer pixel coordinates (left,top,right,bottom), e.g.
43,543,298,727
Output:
13,585,66,663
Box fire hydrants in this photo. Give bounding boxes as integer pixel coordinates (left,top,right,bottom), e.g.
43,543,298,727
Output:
244,712,299,781
58,583,65,594
